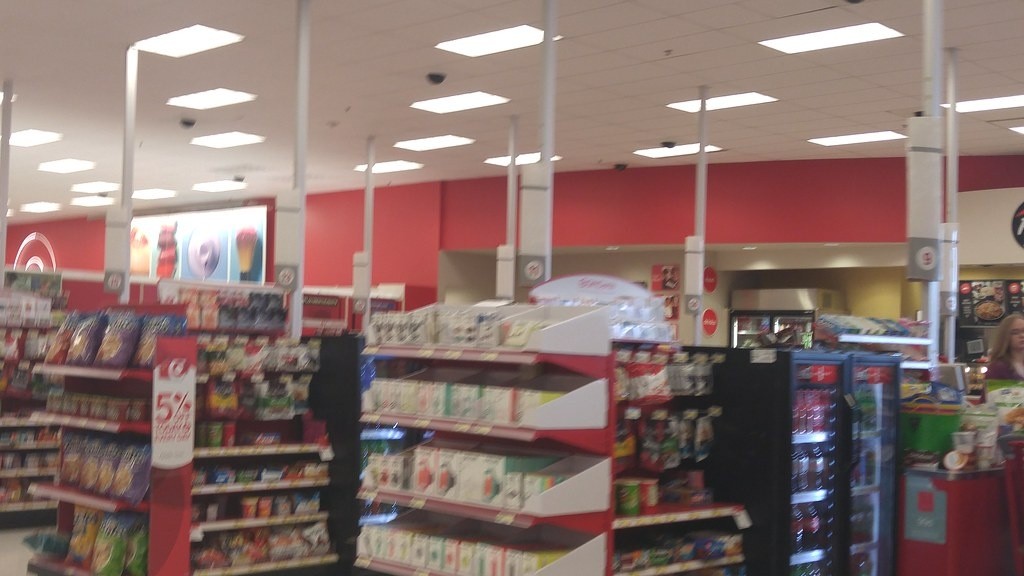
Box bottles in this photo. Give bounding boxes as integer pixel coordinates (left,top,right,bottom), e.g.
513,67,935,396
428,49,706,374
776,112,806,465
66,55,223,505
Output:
792,386,877,576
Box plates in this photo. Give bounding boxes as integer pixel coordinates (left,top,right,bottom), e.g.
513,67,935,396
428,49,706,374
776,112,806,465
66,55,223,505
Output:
973,300,1005,321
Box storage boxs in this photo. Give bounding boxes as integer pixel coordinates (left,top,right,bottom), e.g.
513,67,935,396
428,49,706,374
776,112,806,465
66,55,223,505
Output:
900,383,965,460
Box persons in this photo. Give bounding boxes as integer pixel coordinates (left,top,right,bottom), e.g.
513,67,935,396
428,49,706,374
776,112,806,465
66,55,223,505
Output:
980,313,1024,403
308,336,363,576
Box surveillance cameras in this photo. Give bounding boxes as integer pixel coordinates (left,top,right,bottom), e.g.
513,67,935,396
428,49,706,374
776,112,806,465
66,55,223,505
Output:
662,142,676,148
427,74,445,84
99,193,107,197
915,111,923,117
181,119,196,128
234,177,244,182
615,165,626,170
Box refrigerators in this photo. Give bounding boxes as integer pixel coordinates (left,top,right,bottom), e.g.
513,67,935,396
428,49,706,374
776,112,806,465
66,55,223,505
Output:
682,346,901,576
730,311,814,349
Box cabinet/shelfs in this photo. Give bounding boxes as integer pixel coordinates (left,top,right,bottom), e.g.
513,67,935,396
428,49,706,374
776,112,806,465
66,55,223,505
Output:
814,313,932,381
609,339,796,575
26,301,198,576
176,327,342,576
353,300,617,576
0,271,60,513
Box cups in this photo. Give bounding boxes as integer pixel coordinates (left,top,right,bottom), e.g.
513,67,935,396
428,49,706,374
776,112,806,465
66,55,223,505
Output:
951,431,975,454
